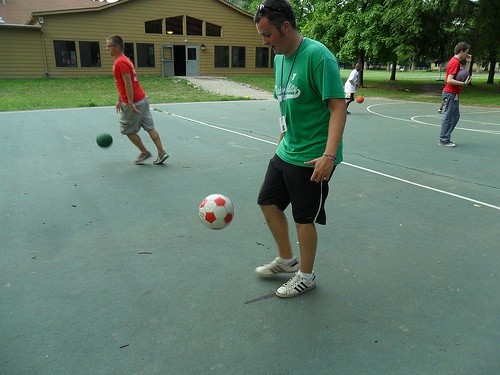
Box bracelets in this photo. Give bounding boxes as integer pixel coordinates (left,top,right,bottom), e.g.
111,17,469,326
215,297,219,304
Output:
463,82,466,85
323,153,336,161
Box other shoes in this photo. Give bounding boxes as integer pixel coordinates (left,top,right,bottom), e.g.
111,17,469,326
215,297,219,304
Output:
439,141,455,146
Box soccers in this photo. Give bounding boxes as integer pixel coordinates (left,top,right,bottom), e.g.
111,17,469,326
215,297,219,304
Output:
198,193,235,230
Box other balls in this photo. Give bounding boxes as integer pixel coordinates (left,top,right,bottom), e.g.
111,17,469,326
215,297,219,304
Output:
357,96,364,103
97,134,113,148
455,69,470,82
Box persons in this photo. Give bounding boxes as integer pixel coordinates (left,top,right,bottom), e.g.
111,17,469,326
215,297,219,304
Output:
344,64,361,115
255,0,347,298
439,42,472,146
105,36,169,164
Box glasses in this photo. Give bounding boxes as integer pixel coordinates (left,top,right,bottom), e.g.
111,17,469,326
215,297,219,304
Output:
107,45,115,48
257,5,290,19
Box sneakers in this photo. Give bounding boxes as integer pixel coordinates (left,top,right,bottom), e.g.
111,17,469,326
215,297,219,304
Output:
153,151,169,165
135,151,151,162
276,272,317,298
255,257,300,278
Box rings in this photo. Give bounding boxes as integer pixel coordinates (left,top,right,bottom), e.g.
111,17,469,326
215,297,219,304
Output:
324,177,326,179
